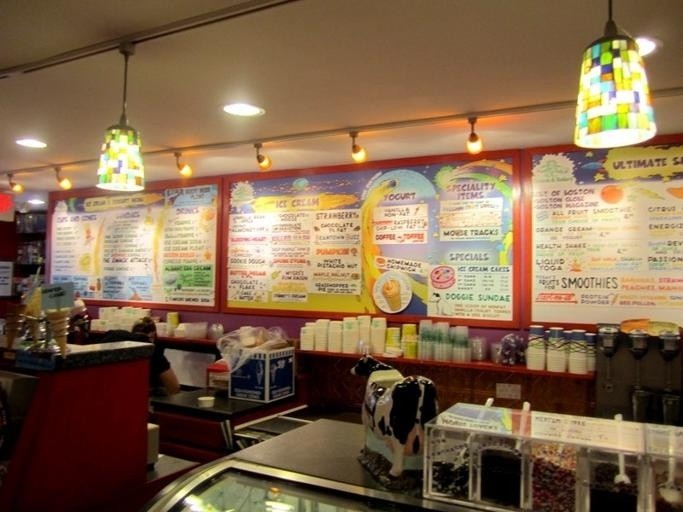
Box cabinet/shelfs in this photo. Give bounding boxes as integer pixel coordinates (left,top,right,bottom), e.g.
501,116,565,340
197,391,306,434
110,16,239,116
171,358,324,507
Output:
13,208,47,297
135,415,508,512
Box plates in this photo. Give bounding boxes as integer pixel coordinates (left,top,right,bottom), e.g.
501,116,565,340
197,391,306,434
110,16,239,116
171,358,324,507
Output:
372,271,413,313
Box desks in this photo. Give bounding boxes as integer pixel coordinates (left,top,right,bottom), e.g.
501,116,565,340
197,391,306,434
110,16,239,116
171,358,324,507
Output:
291,347,598,428
83,321,225,361
150,386,294,451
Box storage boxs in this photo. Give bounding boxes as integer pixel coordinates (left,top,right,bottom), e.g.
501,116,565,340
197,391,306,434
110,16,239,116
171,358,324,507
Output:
227,346,294,404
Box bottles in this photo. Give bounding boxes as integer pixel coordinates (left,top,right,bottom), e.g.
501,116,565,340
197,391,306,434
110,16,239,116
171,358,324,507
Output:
166,312,177,335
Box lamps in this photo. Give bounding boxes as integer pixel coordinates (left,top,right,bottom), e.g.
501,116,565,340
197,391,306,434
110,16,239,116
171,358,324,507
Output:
572,1,660,148
0,112,483,196
94,40,144,192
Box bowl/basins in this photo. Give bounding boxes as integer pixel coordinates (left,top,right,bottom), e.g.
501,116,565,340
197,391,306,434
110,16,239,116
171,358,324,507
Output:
197,396,214,407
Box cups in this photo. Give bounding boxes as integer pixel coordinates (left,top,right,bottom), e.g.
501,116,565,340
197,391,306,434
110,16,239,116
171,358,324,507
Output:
299,315,487,360
91,306,151,333
526,324,596,375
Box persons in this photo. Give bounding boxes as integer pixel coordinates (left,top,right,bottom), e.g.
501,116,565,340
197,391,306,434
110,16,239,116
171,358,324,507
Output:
129,316,182,400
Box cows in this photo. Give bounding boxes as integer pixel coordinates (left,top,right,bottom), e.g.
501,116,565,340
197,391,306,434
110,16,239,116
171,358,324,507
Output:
351,351,440,481
